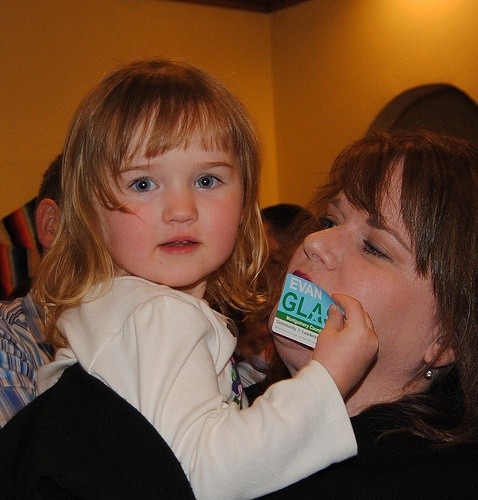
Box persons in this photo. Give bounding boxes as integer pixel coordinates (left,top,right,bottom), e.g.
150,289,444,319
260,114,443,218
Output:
0,58,478,499
